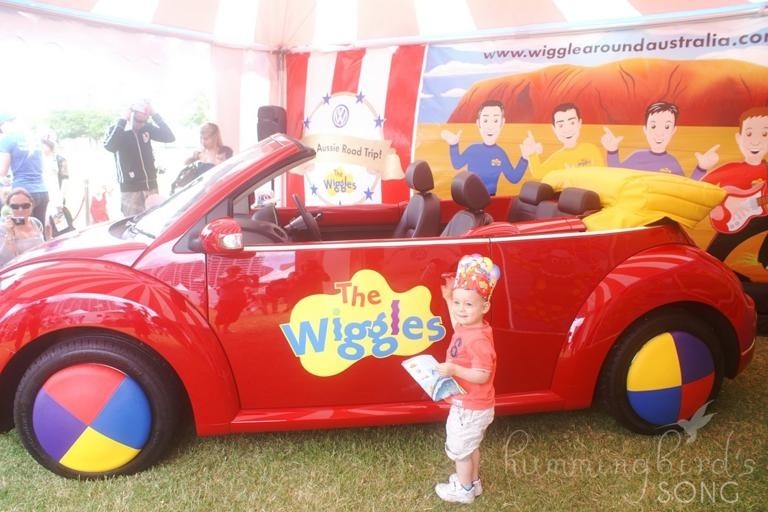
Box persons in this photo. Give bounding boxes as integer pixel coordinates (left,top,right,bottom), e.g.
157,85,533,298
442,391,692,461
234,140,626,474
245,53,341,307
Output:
0,96,233,262
702,106,767,281
440,100,533,196
434,256,501,504
522,102,603,182
600,102,720,182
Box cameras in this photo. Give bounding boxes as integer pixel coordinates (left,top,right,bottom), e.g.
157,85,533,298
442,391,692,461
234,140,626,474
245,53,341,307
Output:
12,217,25,228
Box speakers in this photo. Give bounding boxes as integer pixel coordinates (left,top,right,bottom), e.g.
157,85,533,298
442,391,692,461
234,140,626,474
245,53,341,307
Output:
256,107,285,142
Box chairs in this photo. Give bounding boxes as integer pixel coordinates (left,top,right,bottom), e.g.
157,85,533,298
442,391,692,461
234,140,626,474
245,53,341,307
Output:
392,160,440,238
440,172,493,236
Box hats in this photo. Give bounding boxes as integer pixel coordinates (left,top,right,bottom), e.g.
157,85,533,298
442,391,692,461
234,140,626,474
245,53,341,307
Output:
40,128,58,147
0,112,15,133
451,253,500,302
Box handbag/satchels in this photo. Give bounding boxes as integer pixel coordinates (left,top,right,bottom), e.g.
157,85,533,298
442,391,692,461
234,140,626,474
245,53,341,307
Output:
170,161,214,193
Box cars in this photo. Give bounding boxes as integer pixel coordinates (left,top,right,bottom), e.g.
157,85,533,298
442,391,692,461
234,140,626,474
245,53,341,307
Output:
0,126,759,482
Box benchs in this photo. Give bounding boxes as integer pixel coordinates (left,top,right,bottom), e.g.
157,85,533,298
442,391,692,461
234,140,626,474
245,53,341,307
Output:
508,182,601,222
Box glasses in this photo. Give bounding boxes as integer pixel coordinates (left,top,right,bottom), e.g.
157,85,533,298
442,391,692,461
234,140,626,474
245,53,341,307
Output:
9,203,31,209
133,116,147,123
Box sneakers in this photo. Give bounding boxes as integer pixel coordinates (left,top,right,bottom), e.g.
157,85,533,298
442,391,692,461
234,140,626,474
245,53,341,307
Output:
434,483,475,504
448,474,482,497
